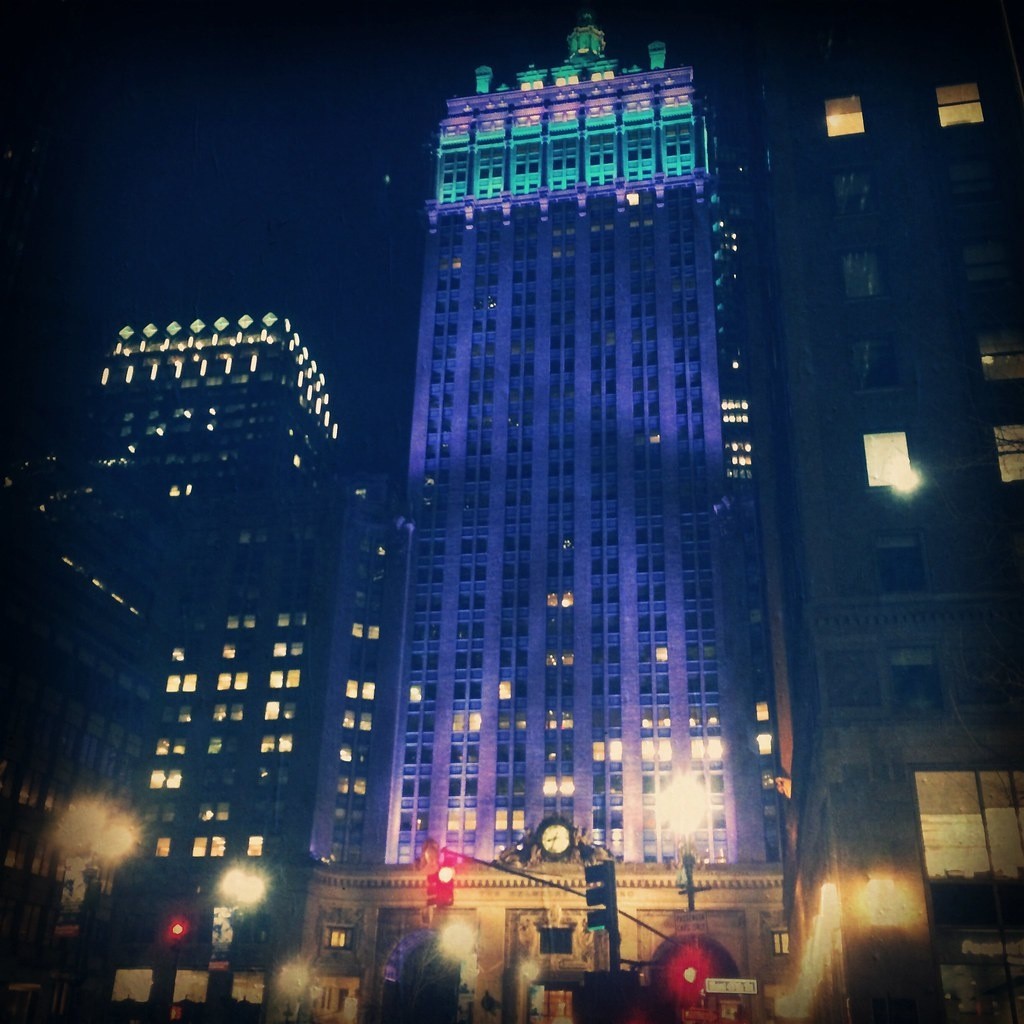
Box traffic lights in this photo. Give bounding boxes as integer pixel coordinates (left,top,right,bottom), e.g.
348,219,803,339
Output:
683,967,699,983
585,864,607,929
436,849,457,905
426,873,435,906
171,922,186,938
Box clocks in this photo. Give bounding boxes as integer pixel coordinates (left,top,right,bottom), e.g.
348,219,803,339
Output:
542,825,570,852
